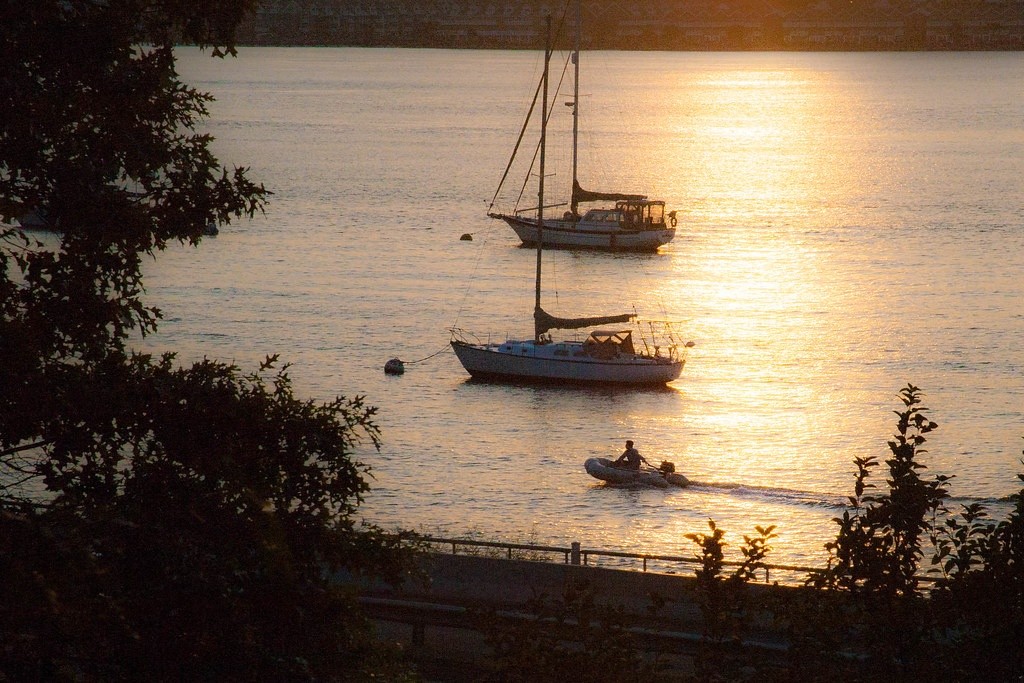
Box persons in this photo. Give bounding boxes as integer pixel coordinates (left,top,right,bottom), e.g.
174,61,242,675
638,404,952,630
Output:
615,440,645,470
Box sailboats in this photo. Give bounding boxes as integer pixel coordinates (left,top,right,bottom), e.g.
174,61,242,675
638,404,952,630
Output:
482,9,678,252
447,16,694,383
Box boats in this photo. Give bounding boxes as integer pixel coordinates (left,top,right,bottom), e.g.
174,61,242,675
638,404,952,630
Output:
583,456,691,488
